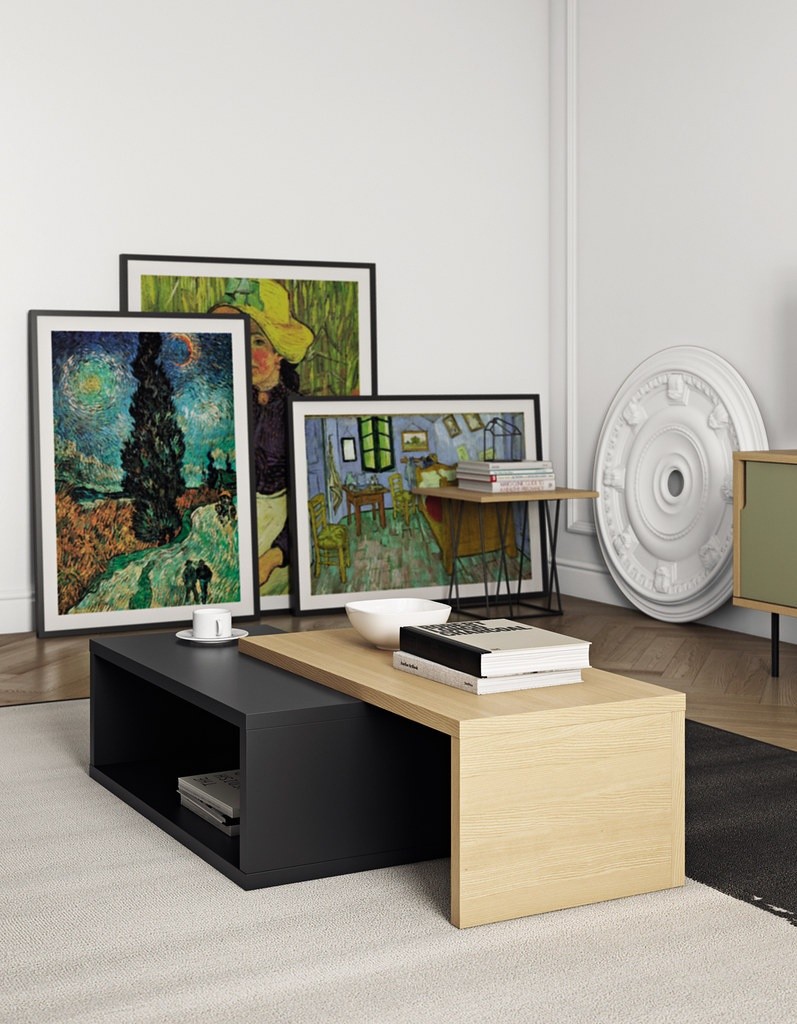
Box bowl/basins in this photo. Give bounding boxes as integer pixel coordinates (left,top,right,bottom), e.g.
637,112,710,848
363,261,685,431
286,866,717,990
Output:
344,596,453,651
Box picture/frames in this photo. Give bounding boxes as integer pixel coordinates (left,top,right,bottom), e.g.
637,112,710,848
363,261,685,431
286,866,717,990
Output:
27,309,260,640
121,252,379,616
285,395,551,616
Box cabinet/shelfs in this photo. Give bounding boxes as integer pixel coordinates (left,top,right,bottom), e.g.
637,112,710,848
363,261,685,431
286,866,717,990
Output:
82,623,449,891
730,448,796,677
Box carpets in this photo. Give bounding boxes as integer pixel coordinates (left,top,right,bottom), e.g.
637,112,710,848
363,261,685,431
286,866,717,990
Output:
0,698,795,1024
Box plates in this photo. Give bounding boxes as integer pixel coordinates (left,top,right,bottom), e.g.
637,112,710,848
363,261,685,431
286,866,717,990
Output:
175,628,249,644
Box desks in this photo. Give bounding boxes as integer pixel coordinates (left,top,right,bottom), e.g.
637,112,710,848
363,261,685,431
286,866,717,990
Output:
238,627,686,931
408,486,600,621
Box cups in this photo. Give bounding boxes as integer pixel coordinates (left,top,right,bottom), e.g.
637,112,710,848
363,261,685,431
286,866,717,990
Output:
192,608,233,639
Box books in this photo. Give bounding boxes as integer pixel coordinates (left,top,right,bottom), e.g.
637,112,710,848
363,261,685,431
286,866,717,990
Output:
456,459,556,493
177,770,241,836
400,618,592,677
393,651,584,696
